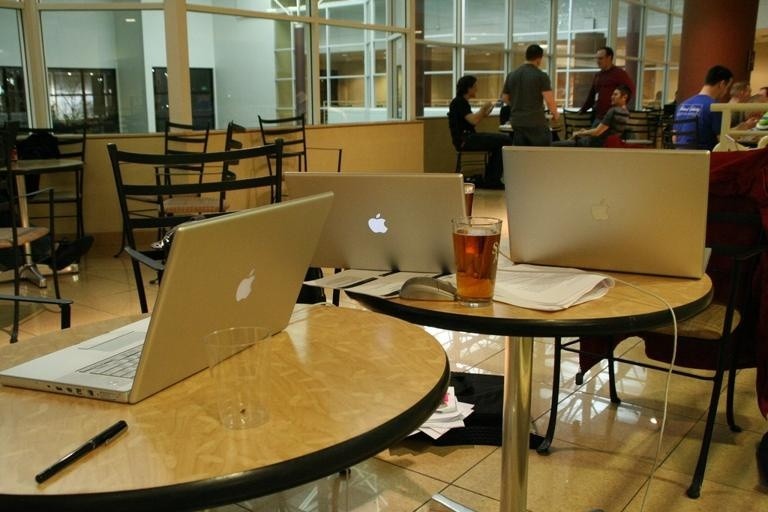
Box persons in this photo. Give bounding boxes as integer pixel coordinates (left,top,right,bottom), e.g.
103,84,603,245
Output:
503,45,560,146
758,87,768,100
726,82,756,131
732,94,767,147
447,75,510,189
0,205,93,273
579,47,636,128
548,86,633,145
671,65,734,149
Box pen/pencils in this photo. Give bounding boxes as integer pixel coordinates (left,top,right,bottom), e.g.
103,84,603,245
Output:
35,420,127,483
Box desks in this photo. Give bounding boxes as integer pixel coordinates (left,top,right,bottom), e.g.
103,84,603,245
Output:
335,258,714,511
0,300,455,511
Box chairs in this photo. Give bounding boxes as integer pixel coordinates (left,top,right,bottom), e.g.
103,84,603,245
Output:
536,241,764,504
447,101,767,188
2,115,343,343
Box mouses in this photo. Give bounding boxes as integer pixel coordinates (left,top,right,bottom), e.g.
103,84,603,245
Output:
400,277,456,301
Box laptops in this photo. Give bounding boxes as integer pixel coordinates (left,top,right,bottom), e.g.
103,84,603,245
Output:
285,171,465,273
0,190,334,404
502,146,712,280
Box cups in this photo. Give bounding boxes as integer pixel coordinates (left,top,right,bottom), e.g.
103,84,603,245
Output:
198,325,273,432
449,214,503,307
